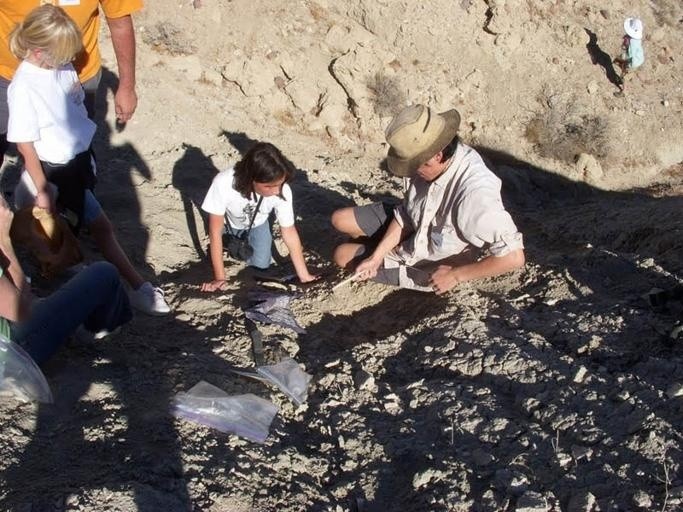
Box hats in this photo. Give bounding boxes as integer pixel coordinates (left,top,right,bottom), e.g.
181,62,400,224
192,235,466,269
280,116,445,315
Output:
624,17,643,41
385,106,461,177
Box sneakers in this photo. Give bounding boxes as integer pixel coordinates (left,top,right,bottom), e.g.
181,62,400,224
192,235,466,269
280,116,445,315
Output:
77,324,122,344
614,93,624,98
129,280,171,316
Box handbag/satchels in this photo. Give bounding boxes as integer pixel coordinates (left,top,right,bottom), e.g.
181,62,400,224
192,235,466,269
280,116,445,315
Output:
225,196,263,260
9,203,83,279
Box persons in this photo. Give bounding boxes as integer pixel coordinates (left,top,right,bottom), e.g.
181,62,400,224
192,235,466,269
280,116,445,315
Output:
0,201,131,359
612,16,645,98
331,103,526,298
6,2,171,318
197,141,317,295
0,0,144,201
